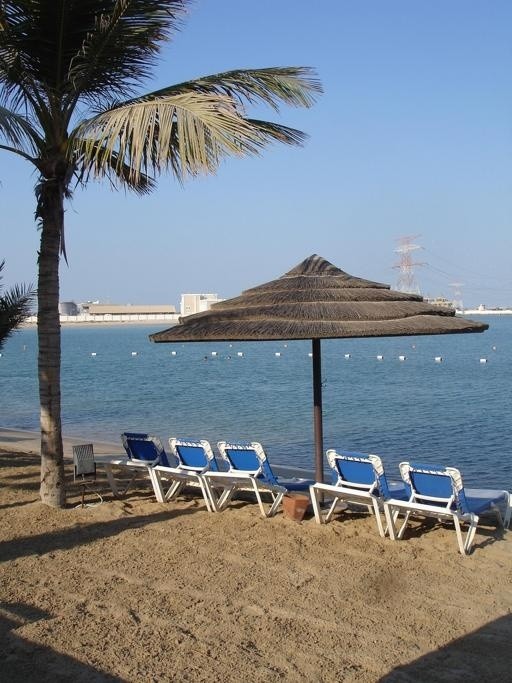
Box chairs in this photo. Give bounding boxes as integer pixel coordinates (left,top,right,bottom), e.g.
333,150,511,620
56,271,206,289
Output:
383,461,510,557
101,432,170,504
202,440,318,518
152,437,218,512
308,448,411,540
